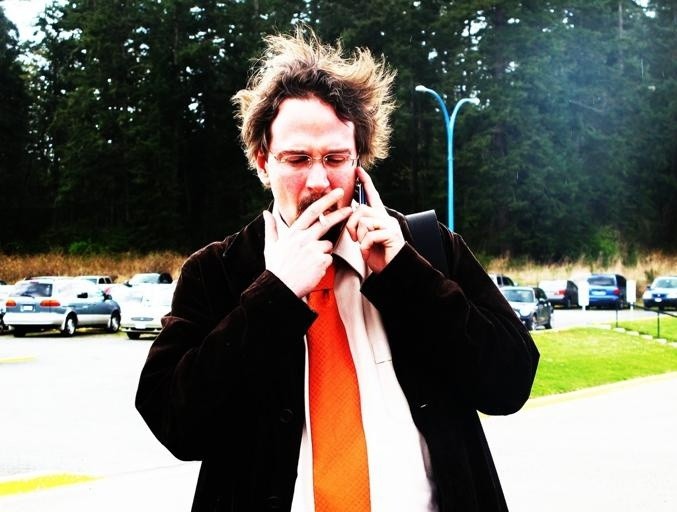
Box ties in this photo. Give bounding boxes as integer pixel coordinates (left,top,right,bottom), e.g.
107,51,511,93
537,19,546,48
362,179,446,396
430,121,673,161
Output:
306,263,372,512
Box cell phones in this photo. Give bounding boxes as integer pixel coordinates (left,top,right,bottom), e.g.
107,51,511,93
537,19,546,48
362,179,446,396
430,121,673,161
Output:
354,182,366,205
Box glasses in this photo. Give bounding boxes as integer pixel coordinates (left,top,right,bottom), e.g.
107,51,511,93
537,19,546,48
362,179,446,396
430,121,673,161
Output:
267,148,362,172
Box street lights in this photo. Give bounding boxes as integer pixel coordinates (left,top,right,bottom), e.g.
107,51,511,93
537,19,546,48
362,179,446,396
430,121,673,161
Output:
415,84,480,231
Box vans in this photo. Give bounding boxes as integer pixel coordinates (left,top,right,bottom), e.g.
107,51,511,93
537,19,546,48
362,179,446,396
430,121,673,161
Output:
586,273,626,308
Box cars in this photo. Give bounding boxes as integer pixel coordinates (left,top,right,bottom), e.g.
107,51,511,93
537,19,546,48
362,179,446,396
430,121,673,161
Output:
642,276,677,310
488,273,578,331
1,273,178,340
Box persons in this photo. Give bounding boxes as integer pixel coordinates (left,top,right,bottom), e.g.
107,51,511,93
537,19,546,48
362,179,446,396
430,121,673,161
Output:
135,19,541,512
